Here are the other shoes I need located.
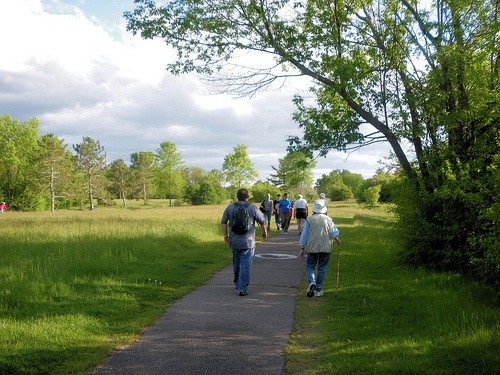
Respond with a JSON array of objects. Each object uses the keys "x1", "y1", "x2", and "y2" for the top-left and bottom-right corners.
[
  {"x1": 238, "y1": 291, "x2": 248, "y2": 296},
  {"x1": 305, "y1": 282, "x2": 316, "y2": 298},
  {"x1": 235, "y1": 287, "x2": 238, "y2": 290},
  {"x1": 315, "y1": 291, "x2": 323, "y2": 297}
]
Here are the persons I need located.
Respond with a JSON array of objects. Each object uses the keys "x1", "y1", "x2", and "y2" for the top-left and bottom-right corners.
[
  {"x1": 279, "y1": 193, "x2": 295, "y2": 235},
  {"x1": 294, "y1": 194, "x2": 309, "y2": 234},
  {"x1": 273, "y1": 194, "x2": 282, "y2": 230},
  {"x1": 319, "y1": 193, "x2": 328, "y2": 208},
  {"x1": 261, "y1": 193, "x2": 274, "y2": 231},
  {"x1": 221, "y1": 188, "x2": 268, "y2": 295},
  {"x1": 300, "y1": 199, "x2": 341, "y2": 298},
  {"x1": 0, "y1": 201, "x2": 7, "y2": 213}
]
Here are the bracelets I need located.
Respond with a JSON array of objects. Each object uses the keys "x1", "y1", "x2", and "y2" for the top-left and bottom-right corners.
[
  {"x1": 223, "y1": 235, "x2": 228, "y2": 237},
  {"x1": 301, "y1": 248, "x2": 304, "y2": 249}
]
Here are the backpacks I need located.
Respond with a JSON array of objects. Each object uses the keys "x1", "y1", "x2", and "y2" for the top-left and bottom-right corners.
[{"x1": 230, "y1": 201, "x2": 252, "y2": 235}]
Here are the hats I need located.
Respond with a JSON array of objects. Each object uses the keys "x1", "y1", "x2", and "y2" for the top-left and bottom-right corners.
[
  {"x1": 319, "y1": 192, "x2": 325, "y2": 198},
  {"x1": 311, "y1": 200, "x2": 327, "y2": 213}
]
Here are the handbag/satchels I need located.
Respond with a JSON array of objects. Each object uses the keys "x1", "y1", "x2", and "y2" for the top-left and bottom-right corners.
[{"x1": 259, "y1": 200, "x2": 266, "y2": 212}]
